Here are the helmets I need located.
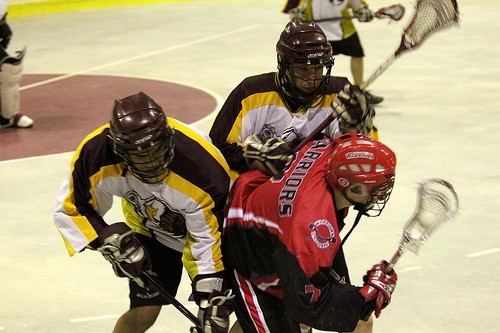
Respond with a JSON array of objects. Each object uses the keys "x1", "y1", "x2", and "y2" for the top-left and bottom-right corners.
[
  {"x1": 275, "y1": 21, "x2": 334, "y2": 109},
  {"x1": 325, "y1": 131, "x2": 397, "y2": 217},
  {"x1": 108, "y1": 91, "x2": 175, "y2": 183}
]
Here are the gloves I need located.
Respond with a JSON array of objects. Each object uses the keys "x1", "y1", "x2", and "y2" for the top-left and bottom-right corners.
[
  {"x1": 357, "y1": 6, "x2": 374, "y2": 21},
  {"x1": 99, "y1": 221, "x2": 152, "y2": 278},
  {"x1": 361, "y1": 259, "x2": 397, "y2": 318},
  {"x1": 190, "y1": 270, "x2": 236, "y2": 332},
  {"x1": 244, "y1": 133, "x2": 291, "y2": 179}
]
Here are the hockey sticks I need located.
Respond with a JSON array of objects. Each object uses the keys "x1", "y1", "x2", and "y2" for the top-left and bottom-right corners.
[
  {"x1": 289, "y1": 0, "x2": 459, "y2": 154},
  {"x1": 312, "y1": 4, "x2": 404, "y2": 22},
  {"x1": 140, "y1": 271, "x2": 201, "y2": 327},
  {"x1": 385, "y1": 177, "x2": 460, "y2": 273}
]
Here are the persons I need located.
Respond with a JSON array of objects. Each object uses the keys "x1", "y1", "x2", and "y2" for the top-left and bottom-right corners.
[
  {"x1": 220, "y1": 134, "x2": 397, "y2": 333},
  {"x1": 281, "y1": 0, "x2": 384, "y2": 104},
  {"x1": 0, "y1": 0, "x2": 34, "y2": 128},
  {"x1": 209, "y1": 18, "x2": 379, "y2": 333},
  {"x1": 53, "y1": 92, "x2": 240, "y2": 333}
]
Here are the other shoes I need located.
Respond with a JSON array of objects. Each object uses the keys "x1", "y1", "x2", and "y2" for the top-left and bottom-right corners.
[
  {"x1": 13, "y1": 113, "x2": 34, "y2": 126},
  {"x1": 365, "y1": 90, "x2": 384, "y2": 103}
]
